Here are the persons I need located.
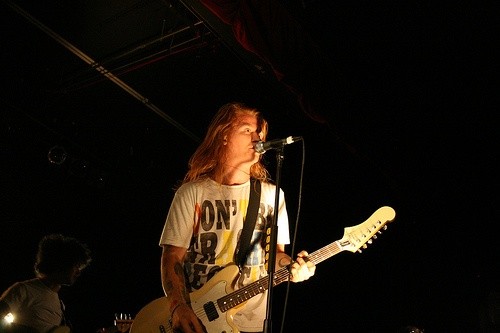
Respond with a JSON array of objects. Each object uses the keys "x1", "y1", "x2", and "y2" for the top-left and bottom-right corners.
[
  {"x1": 158, "y1": 101, "x2": 316, "y2": 333},
  {"x1": 0, "y1": 234, "x2": 92, "y2": 333}
]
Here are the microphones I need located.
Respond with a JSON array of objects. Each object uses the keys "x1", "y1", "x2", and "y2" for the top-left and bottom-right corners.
[{"x1": 254, "y1": 137, "x2": 300, "y2": 154}]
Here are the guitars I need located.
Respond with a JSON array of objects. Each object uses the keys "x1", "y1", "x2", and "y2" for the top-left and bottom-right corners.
[
  {"x1": 50, "y1": 312, "x2": 134, "y2": 333},
  {"x1": 129, "y1": 205, "x2": 397, "y2": 333}
]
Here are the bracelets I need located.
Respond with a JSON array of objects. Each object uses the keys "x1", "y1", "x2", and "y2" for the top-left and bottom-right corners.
[{"x1": 168, "y1": 302, "x2": 188, "y2": 320}]
